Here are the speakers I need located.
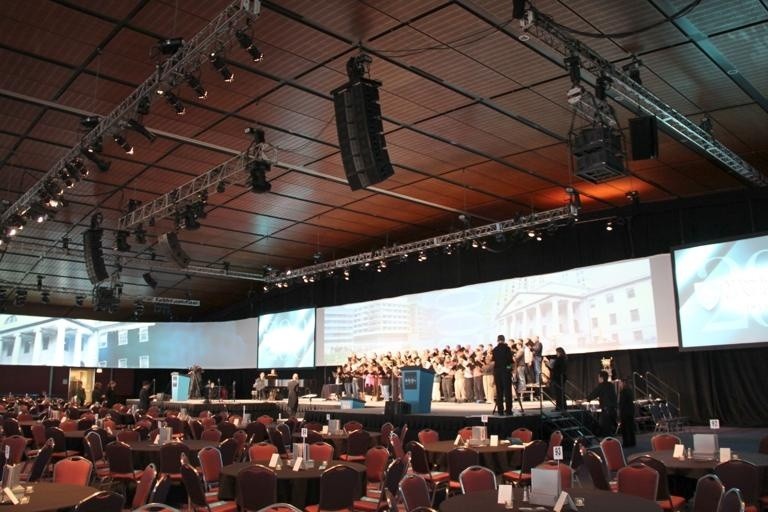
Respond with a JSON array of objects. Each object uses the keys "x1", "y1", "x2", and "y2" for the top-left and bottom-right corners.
[
  {"x1": 628, "y1": 115, "x2": 659, "y2": 160},
  {"x1": 385, "y1": 401, "x2": 411, "y2": 415}
]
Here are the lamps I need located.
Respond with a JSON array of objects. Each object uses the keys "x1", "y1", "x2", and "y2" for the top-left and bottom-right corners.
[{"x1": 260, "y1": 206, "x2": 580, "y2": 294}]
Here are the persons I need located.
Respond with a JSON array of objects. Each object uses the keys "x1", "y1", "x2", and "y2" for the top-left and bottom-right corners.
[
  {"x1": 138, "y1": 380, "x2": 157, "y2": 411},
  {"x1": 285, "y1": 373, "x2": 304, "y2": 417},
  {"x1": 615, "y1": 376, "x2": 637, "y2": 449},
  {"x1": 574, "y1": 370, "x2": 619, "y2": 441},
  {"x1": 252, "y1": 371, "x2": 268, "y2": 400},
  {"x1": 545, "y1": 347, "x2": 570, "y2": 412},
  {"x1": 76, "y1": 380, "x2": 86, "y2": 406},
  {"x1": 104, "y1": 379, "x2": 118, "y2": 409},
  {"x1": 92, "y1": 381, "x2": 106, "y2": 406},
  {"x1": 332, "y1": 333, "x2": 544, "y2": 417}
]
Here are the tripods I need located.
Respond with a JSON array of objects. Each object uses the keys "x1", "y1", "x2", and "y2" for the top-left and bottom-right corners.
[{"x1": 189, "y1": 374, "x2": 201, "y2": 398}]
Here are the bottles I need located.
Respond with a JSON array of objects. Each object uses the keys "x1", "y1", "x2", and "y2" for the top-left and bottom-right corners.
[
  {"x1": 687, "y1": 448, "x2": 691, "y2": 459},
  {"x1": 523, "y1": 485, "x2": 531, "y2": 501}
]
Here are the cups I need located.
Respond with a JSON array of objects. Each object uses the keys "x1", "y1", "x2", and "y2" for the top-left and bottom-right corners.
[
  {"x1": 575, "y1": 497, "x2": 585, "y2": 505},
  {"x1": 609, "y1": 480, "x2": 619, "y2": 494},
  {"x1": 505, "y1": 499, "x2": 514, "y2": 509}
]
